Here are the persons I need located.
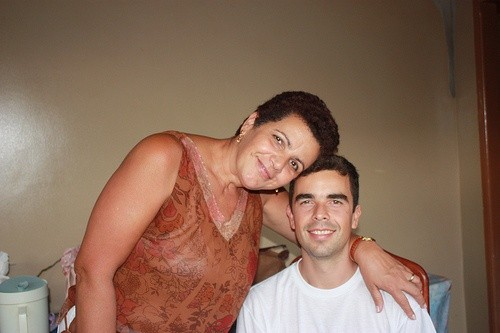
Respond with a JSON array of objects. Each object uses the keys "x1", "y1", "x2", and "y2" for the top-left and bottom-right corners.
[
  {"x1": 58, "y1": 91, "x2": 428, "y2": 333},
  {"x1": 235, "y1": 153, "x2": 438, "y2": 333}
]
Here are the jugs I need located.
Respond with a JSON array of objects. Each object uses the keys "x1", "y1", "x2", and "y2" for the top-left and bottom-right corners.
[{"x1": 0, "y1": 276, "x2": 49, "y2": 333}]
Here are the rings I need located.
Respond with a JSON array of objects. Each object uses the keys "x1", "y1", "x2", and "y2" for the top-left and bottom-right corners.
[{"x1": 409, "y1": 273, "x2": 415, "y2": 282}]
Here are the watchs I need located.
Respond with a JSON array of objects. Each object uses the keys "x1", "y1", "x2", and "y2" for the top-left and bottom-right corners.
[{"x1": 349, "y1": 235, "x2": 377, "y2": 262}]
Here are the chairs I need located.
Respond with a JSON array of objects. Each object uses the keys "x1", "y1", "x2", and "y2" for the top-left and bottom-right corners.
[{"x1": 290, "y1": 249, "x2": 430, "y2": 315}]
